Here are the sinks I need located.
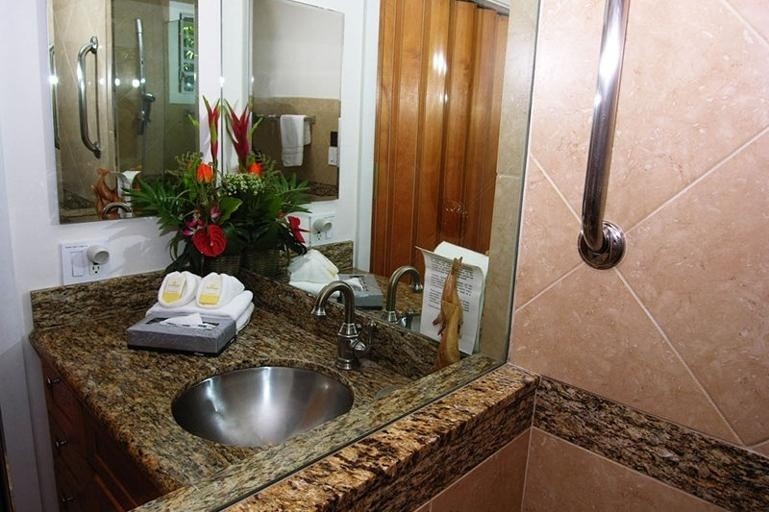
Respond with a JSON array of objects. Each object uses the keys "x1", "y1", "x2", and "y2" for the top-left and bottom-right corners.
[
  {"x1": 171, "y1": 361, "x2": 353, "y2": 448},
  {"x1": 397, "y1": 314, "x2": 425, "y2": 333}
]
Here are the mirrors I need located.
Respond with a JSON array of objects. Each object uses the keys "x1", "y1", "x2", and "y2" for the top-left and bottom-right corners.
[
  {"x1": 46, "y1": 1, "x2": 200, "y2": 225},
  {"x1": 220, "y1": 0, "x2": 544, "y2": 361},
  {"x1": 243, "y1": 2, "x2": 343, "y2": 207}
]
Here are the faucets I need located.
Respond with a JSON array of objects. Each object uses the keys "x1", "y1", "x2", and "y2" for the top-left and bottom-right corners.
[
  {"x1": 101, "y1": 203, "x2": 132, "y2": 219},
  {"x1": 379, "y1": 266, "x2": 424, "y2": 330},
  {"x1": 311, "y1": 280, "x2": 376, "y2": 372}
]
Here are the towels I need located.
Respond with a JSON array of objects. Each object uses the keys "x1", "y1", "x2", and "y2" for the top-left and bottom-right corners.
[
  {"x1": 146, "y1": 273, "x2": 255, "y2": 337},
  {"x1": 290, "y1": 250, "x2": 343, "y2": 303},
  {"x1": 276, "y1": 113, "x2": 311, "y2": 169}
]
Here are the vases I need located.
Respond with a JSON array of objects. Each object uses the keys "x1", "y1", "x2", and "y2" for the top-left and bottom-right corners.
[
  {"x1": 245, "y1": 245, "x2": 287, "y2": 277},
  {"x1": 184, "y1": 245, "x2": 242, "y2": 281}
]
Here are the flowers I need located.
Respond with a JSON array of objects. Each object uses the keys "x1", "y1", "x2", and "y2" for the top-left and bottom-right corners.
[
  {"x1": 121, "y1": 94, "x2": 247, "y2": 272},
  {"x1": 222, "y1": 102, "x2": 312, "y2": 258}
]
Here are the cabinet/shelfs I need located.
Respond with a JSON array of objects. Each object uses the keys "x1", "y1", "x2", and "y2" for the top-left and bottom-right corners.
[{"x1": 40, "y1": 359, "x2": 162, "y2": 511}]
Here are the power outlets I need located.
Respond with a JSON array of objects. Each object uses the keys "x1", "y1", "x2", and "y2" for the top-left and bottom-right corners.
[
  {"x1": 86, "y1": 238, "x2": 111, "y2": 281},
  {"x1": 308, "y1": 217, "x2": 326, "y2": 244}
]
